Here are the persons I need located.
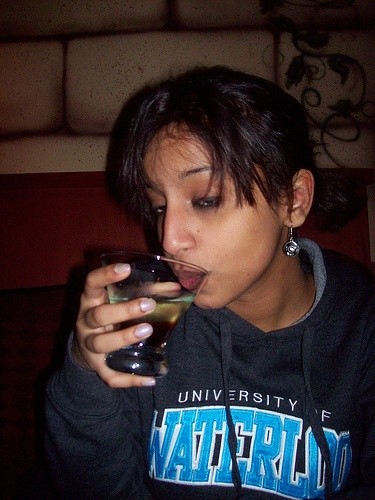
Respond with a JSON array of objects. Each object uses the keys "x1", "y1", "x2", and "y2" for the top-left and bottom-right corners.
[{"x1": 42, "y1": 60, "x2": 375, "y2": 500}]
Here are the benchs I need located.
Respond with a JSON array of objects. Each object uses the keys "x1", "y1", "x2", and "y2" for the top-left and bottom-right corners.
[{"x1": 1, "y1": 166, "x2": 375, "y2": 500}]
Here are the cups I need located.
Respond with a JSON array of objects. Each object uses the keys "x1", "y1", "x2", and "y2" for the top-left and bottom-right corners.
[{"x1": 98, "y1": 251, "x2": 209, "y2": 379}]
